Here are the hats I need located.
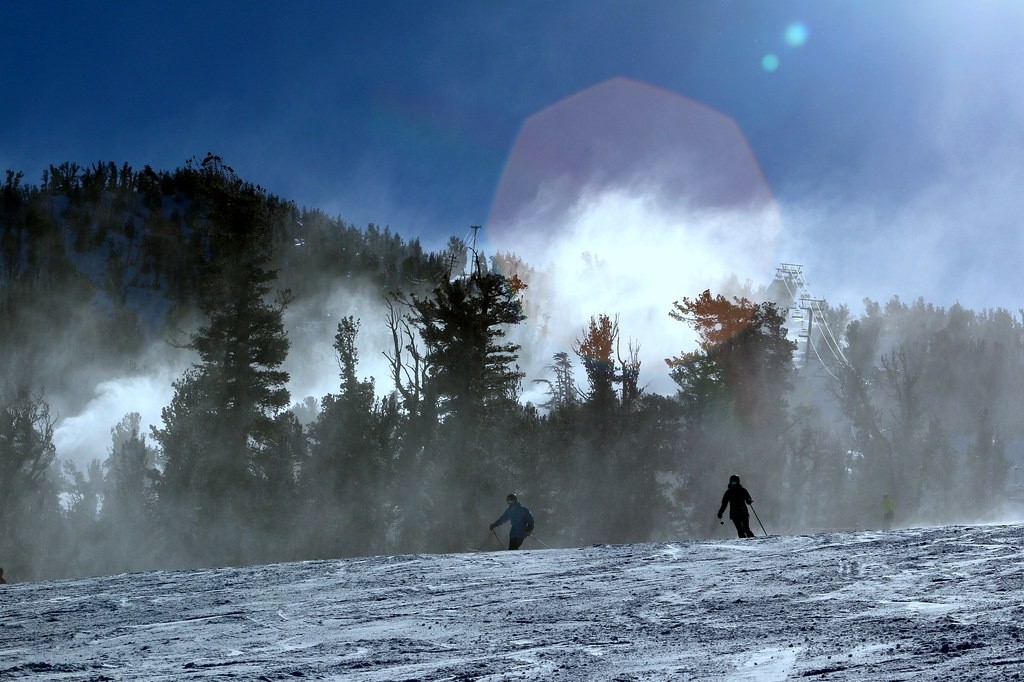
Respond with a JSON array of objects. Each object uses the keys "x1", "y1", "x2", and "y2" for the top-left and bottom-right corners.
[
  {"x1": 730, "y1": 475, "x2": 740, "y2": 485},
  {"x1": 507, "y1": 494, "x2": 517, "y2": 501}
]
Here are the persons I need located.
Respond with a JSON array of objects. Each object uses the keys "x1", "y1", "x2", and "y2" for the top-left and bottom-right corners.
[
  {"x1": 718, "y1": 474, "x2": 754, "y2": 538},
  {"x1": 490, "y1": 494, "x2": 535, "y2": 550},
  {"x1": 881, "y1": 493, "x2": 895, "y2": 531},
  {"x1": 0, "y1": 568, "x2": 6, "y2": 584}
]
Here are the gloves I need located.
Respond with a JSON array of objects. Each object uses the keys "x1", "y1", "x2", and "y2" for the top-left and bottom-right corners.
[
  {"x1": 489, "y1": 523, "x2": 497, "y2": 531},
  {"x1": 746, "y1": 499, "x2": 754, "y2": 505},
  {"x1": 527, "y1": 530, "x2": 531, "y2": 536},
  {"x1": 718, "y1": 513, "x2": 722, "y2": 519}
]
